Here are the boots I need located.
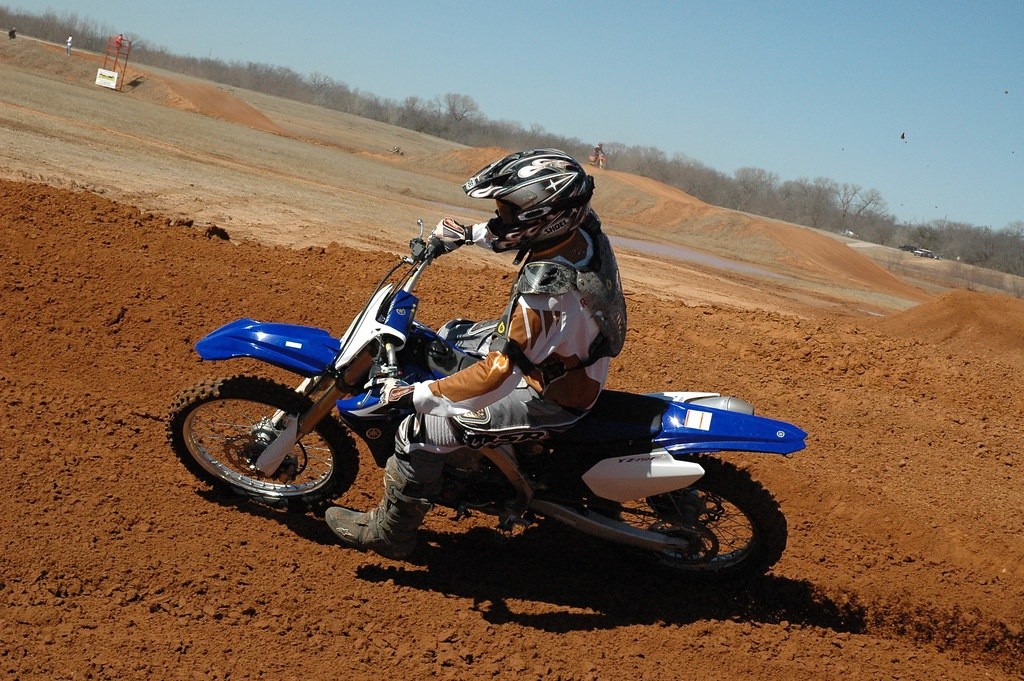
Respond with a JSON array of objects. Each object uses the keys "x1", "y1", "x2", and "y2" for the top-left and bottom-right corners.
[{"x1": 325, "y1": 454, "x2": 431, "y2": 552}]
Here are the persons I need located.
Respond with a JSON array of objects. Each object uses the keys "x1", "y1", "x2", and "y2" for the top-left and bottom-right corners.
[
  {"x1": 592, "y1": 142, "x2": 604, "y2": 165},
  {"x1": 66, "y1": 34, "x2": 73, "y2": 54},
  {"x1": 8, "y1": 26, "x2": 17, "y2": 40},
  {"x1": 116, "y1": 34, "x2": 124, "y2": 47},
  {"x1": 325, "y1": 149, "x2": 628, "y2": 548}
]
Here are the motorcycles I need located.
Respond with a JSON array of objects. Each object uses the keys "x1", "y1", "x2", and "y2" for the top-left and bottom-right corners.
[
  {"x1": 163, "y1": 220, "x2": 808, "y2": 590},
  {"x1": 589, "y1": 153, "x2": 608, "y2": 170}
]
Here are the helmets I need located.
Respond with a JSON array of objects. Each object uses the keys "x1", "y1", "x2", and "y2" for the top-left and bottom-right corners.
[{"x1": 462, "y1": 148, "x2": 593, "y2": 253}]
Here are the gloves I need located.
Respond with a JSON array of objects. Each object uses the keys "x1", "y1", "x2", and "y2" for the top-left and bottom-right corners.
[
  {"x1": 427, "y1": 217, "x2": 474, "y2": 254},
  {"x1": 363, "y1": 377, "x2": 414, "y2": 410}
]
